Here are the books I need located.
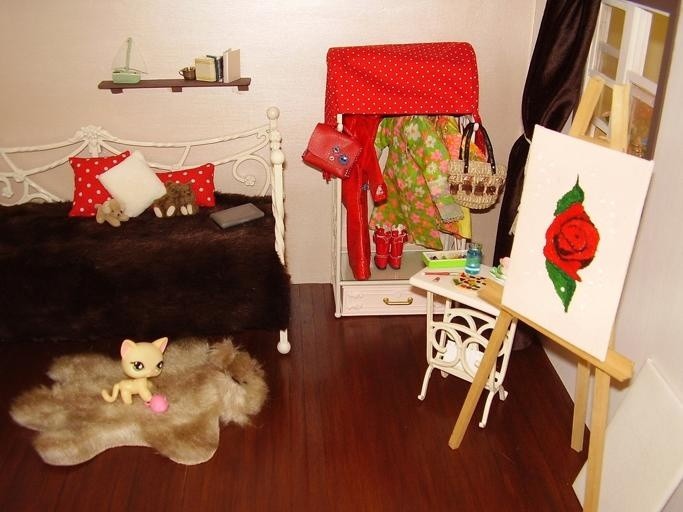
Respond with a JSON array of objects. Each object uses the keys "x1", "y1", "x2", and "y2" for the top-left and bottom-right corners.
[
  {"x1": 194, "y1": 57, "x2": 216, "y2": 83},
  {"x1": 222, "y1": 47, "x2": 241, "y2": 84},
  {"x1": 206, "y1": 55, "x2": 223, "y2": 83}
]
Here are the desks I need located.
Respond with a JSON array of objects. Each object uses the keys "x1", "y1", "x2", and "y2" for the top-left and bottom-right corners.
[{"x1": 407, "y1": 263, "x2": 519, "y2": 428}]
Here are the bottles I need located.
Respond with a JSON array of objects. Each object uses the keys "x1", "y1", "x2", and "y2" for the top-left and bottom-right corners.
[{"x1": 465, "y1": 242, "x2": 482, "y2": 274}]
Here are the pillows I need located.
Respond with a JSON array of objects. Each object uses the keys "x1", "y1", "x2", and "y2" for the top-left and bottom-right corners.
[{"x1": 68, "y1": 149, "x2": 215, "y2": 218}]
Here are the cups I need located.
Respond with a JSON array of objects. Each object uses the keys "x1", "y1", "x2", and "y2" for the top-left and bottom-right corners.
[{"x1": 178, "y1": 66, "x2": 196, "y2": 80}]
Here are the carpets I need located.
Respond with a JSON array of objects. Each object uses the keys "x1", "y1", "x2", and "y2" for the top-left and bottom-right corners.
[{"x1": 6, "y1": 336, "x2": 269, "y2": 467}]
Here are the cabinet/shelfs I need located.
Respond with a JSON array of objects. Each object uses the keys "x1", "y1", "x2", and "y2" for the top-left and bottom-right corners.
[{"x1": 329, "y1": 114, "x2": 479, "y2": 318}]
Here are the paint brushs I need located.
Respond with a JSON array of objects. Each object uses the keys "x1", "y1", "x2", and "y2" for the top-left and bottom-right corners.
[{"x1": 424, "y1": 272, "x2": 461, "y2": 276}]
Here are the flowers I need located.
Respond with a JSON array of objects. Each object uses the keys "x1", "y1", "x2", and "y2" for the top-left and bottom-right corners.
[{"x1": 543, "y1": 173, "x2": 600, "y2": 313}]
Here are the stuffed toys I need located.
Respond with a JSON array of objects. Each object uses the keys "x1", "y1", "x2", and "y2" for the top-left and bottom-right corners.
[
  {"x1": 93, "y1": 198, "x2": 130, "y2": 228},
  {"x1": 152, "y1": 180, "x2": 181, "y2": 218},
  {"x1": 174, "y1": 182, "x2": 199, "y2": 216}
]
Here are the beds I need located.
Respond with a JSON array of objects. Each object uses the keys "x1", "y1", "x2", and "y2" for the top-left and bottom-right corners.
[{"x1": 0, "y1": 107, "x2": 293, "y2": 354}]
